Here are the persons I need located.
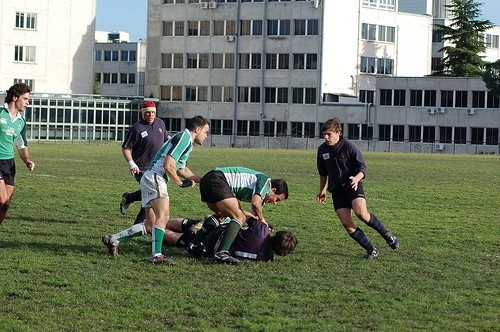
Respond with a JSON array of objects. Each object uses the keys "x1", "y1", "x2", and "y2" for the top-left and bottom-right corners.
[
  {"x1": 317, "y1": 119, "x2": 397, "y2": 258},
  {"x1": 164, "y1": 211, "x2": 298, "y2": 263},
  {"x1": 199, "y1": 167, "x2": 288, "y2": 265},
  {"x1": 0, "y1": 83, "x2": 36, "y2": 225},
  {"x1": 120, "y1": 101, "x2": 172, "y2": 224},
  {"x1": 103, "y1": 115, "x2": 211, "y2": 264}
]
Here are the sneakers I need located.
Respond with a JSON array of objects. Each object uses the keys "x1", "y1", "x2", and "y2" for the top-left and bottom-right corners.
[
  {"x1": 366, "y1": 247, "x2": 377, "y2": 258},
  {"x1": 103, "y1": 235, "x2": 119, "y2": 256},
  {"x1": 213, "y1": 252, "x2": 240, "y2": 264},
  {"x1": 187, "y1": 242, "x2": 212, "y2": 257},
  {"x1": 386, "y1": 234, "x2": 399, "y2": 249},
  {"x1": 120, "y1": 193, "x2": 130, "y2": 214},
  {"x1": 149, "y1": 255, "x2": 176, "y2": 266}
]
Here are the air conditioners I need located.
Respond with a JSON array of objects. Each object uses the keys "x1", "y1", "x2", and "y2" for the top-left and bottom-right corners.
[
  {"x1": 200, "y1": 2, "x2": 208, "y2": 8},
  {"x1": 427, "y1": 108, "x2": 436, "y2": 114},
  {"x1": 209, "y1": 2, "x2": 217, "y2": 8},
  {"x1": 225, "y1": 36, "x2": 235, "y2": 42},
  {"x1": 465, "y1": 108, "x2": 475, "y2": 115},
  {"x1": 310, "y1": 0, "x2": 319, "y2": 8},
  {"x1": 437, "y1": 107, "x2": 446, "y2": 113},
  {"x1": 435, "y1": 144, "x2": 444, "y2": 150}
]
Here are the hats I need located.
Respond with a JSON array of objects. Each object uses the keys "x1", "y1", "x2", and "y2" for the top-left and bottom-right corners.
[{"x1": 140, "y1": 102, "x2": 156, "y2": 120}]
